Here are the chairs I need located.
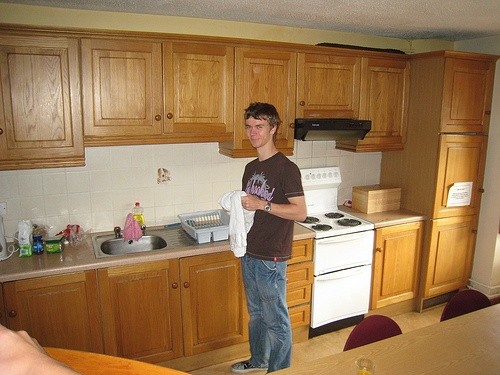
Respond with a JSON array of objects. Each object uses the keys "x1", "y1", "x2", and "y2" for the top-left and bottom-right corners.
[
  {"x1": 440, "y1": 289, "x2": 491, "y2": 322},
  {"x1": 343, "y1": 314, "x2": 403, "y2": 352}
]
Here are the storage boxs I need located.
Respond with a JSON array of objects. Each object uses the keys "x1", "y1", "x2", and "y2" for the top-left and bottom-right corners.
[{"x1": 352, "y1": 184, "x2": 401, "y2": 215}]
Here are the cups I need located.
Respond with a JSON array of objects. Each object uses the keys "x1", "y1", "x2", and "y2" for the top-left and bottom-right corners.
[{"x1": 355, "y1": 358, "x2": 375, "y2": 375}]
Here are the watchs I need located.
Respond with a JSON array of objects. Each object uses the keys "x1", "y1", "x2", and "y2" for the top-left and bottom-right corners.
[{"x1": 264, "y1": 202, "x2": 272, "y2": 212}]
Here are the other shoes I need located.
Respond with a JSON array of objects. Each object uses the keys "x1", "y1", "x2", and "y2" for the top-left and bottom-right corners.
[{"x1": 231, "y1": 361, "x2": 269, "y2": 372}]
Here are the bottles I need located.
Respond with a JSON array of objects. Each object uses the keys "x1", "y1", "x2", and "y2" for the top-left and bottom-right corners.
[
  {"x1": 32, "y1": 225, "x2": 44, "y2": 254},
  {"x1": 131, "y1": 202, "x2": 144, "y2": 226}
]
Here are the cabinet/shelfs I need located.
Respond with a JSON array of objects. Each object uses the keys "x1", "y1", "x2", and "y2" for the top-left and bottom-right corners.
[
  {"x1": 417, "y1": 214, "x2": 479, "y2": 312},
  {"x1": 380, "y1": 132, "x2": 489, "y2": 218},
  {"x1": 0, "y1": 268, "x2": 102, "y2": 356},
  {"x1": 371, "y1": 221, "x2": 426, "y2": 311},
  {"x1": 77, "y1": 26, "x2": 234, "y2": 147},
  {"x1": 219, "y1": 37, "x2": 295, "y2": 159},
  {"x1": 297, "y1": 45, "x2": 359, "y2": 121},
  {"x1": 335, "y1": 44, "x2": 409, "y2": 153},
  {"x1": 97, "y1": 252, "x2": 251, "y2": 372},
  {"x1": 407, "y1": 50, "x2": 500, "y2": 132},
  {"x1": 0, "y1": 21, "x2": 86, "y2": 171},
  {"x1": 285, "y1": 236, "x2": 315, "y2": 343}
]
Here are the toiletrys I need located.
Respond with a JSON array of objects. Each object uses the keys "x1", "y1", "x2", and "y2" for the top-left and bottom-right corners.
[{"x1": 131, "y1": 201, "x2": 145, "y2": 226}]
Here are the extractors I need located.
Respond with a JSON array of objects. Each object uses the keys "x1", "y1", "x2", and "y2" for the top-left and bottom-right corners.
[{"x1": 295, "y1": 117, "x2": 372, "y2": 141}]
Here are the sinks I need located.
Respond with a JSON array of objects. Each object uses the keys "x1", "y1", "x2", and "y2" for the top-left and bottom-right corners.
[{"x1": 91, "y1": 226, "x2": 175, "y2": 259}]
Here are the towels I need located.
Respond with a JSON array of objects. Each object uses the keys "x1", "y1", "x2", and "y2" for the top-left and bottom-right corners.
[
  {"x1": 216, "y1": 190, "x2": 256, "y2": 258},
  {"x1": 122, "y1": 213, "x2": 144, "y2": 241}
]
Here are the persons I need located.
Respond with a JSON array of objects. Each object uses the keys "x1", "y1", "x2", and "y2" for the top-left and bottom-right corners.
[
  {"x1": 231, "y1": 102, "x2": 307, "y2": 375},
  {"x1": 0, "y1": 323, "x2": 81, "y2": 375}
]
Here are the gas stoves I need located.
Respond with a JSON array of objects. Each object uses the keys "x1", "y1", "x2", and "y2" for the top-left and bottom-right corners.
[{"x1": 295, "y1": 167, "x2": 375, "y2": 239}]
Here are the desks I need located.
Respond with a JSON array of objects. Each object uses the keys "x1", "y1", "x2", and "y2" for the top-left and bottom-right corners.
[{"x1": 264, "y1": 304, "x2": 500, "y2": 375}]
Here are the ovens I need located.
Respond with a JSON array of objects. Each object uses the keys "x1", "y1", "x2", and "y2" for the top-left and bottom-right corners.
[{"x1": 309, "y1": 230, "x2": 375, "y2": 338}]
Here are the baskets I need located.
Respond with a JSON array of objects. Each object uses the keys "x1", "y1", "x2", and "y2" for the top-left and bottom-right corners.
[{"x1": 352, "y1": 186, "x2": 402, "y2": 214}]
